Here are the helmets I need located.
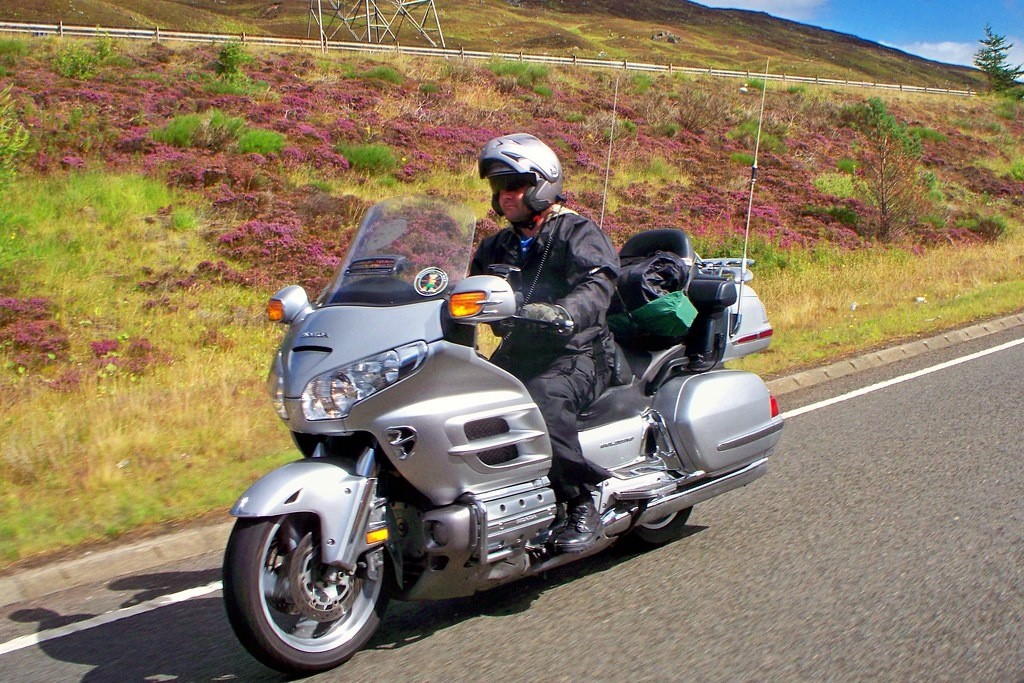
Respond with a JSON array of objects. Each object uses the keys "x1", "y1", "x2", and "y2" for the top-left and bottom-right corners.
[{"x1": 478, "y1": 132, "x2": 564, "y2": 218}]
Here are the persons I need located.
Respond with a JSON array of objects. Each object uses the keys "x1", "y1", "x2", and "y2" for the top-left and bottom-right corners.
[{"x1": 467, "y1": 133, "x2": 620, "y2": 554}]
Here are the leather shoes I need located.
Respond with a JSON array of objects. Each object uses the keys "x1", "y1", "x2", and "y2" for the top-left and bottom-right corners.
[{"x1": 554, "y1": 496, "x2": 606, "y2": 553}]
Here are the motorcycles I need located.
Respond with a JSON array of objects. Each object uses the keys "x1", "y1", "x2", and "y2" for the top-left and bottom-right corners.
[{"x1": 220, "y1": 195, "x2": 784, "y2": 679}]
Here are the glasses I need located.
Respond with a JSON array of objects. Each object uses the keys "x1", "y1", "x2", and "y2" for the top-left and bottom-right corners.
[{"x1": 488, "y1": 173, "x2": 547, "y2": 192}]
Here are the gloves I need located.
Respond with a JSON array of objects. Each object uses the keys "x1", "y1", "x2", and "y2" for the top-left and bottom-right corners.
[{"x1": 520, "y1": 302, "x2": 575, "y2": 337}]
